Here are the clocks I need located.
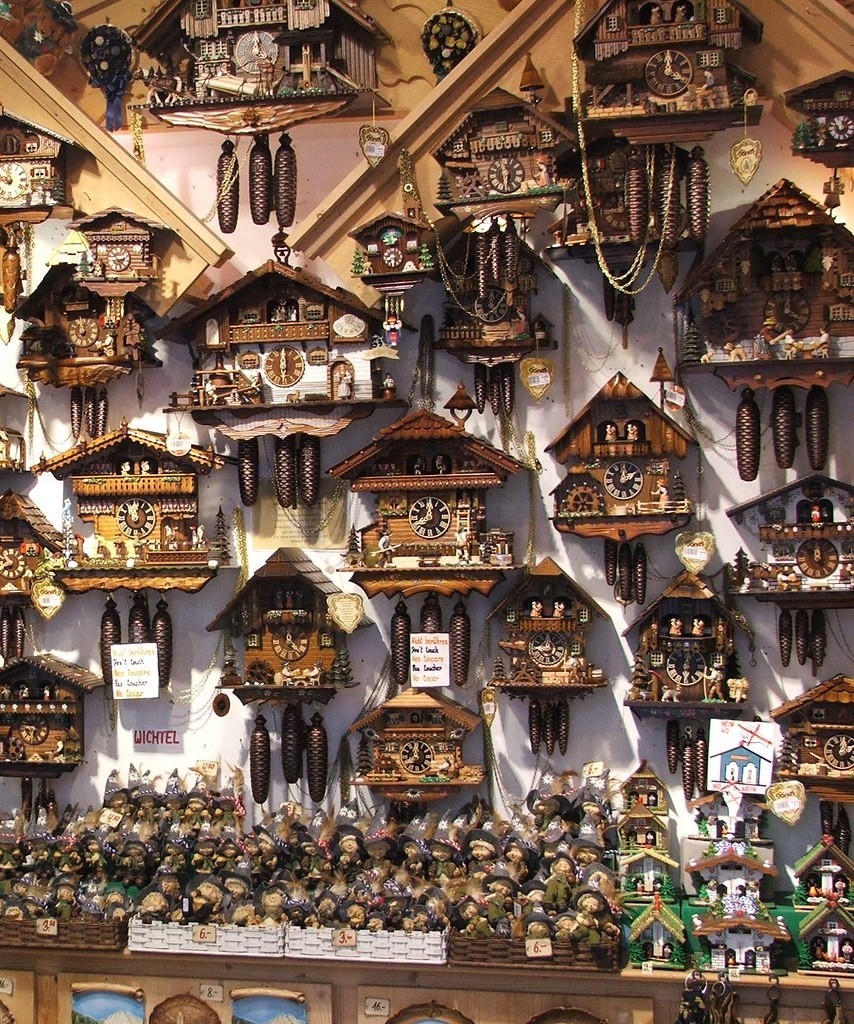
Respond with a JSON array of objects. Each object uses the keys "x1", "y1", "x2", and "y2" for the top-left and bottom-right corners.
[{"x1": 0, "y1": 0, "x2": 854, "y2": 858}]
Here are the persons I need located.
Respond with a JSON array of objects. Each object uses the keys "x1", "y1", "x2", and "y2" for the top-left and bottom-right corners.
[{"x1": 0, "y1": 0, "x2": 854, "y2": 980}]
